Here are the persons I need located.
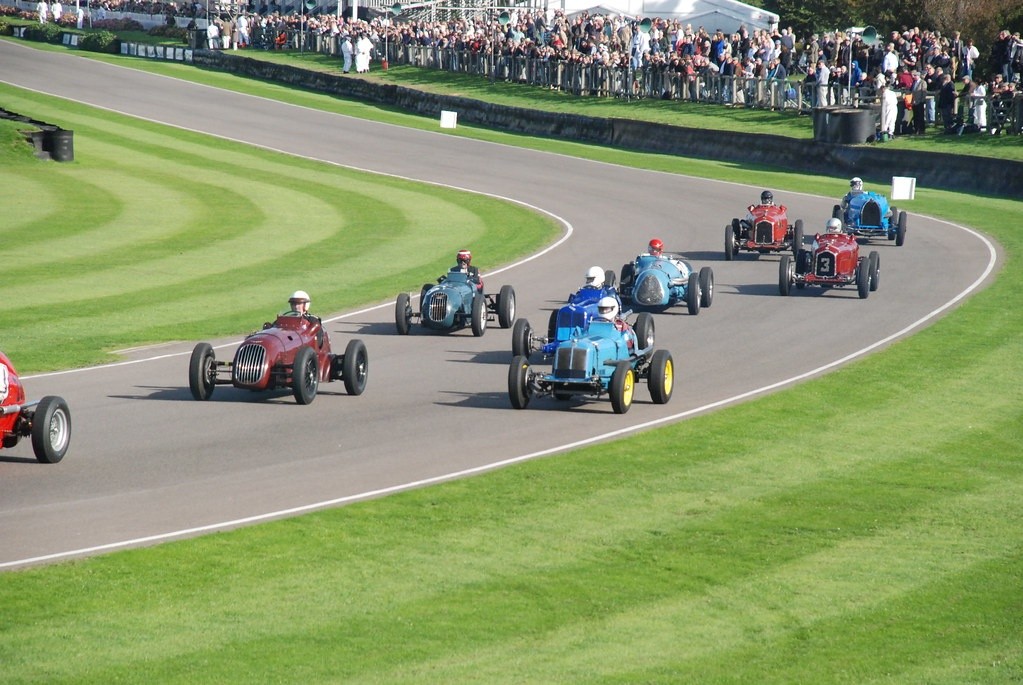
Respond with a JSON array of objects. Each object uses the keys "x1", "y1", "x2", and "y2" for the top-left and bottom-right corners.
[
  {"x1": 77, "y1": 6, "x2": 85, "y2": 30},
  {"x1": 826, "y1": 218, "x2": 842, "y2": 234},
  {"x1": 36, "y1": 0, "x2": 49, "y2": 24},
  {"x1": 841, "y1": 177, "x2": 868, "y2": 212},
  {"x1": 648, "y1": 239, "x2": 664, "y2": 257},
  {"x1": 598, "y1": 297, "x2": 633, "y2": 349},
  {"x1": 51, "y1": 0, "x2": 63, "y2": 25},
  {"x1": 451, "y1": 250, "x2": 483, "y2": 290},
  {"x1": 585, "y1": 266, "x2": 605, "y2": 290},
  {"x1": 760, "y1": 190, "x2": 776, "y2": 206},
  {"x1": 288, "y1": 290, "x2": 324, "y2": 349},
  {"x1": 64, "y1": 0, "x2": 1023, "y2": 140}
]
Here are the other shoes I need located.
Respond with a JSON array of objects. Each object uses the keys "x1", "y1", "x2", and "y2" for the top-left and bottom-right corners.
[{"x1": 344, "y1": 71, "x2": 350, "y2": 74}]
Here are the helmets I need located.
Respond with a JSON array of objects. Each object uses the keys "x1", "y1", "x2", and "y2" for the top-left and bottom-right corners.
[
  {"x1": 850, "y1": 177, "x2": 862, "y2": 191},
  {"x1": 288, "y1": 290, "x2": 311, "y2": 312},
  {"x1": 598, "y1": 296, "x2": 619, "y2": 320},
  {"x1": 585, "y1": 266, "x2": 605, "y2": 286},
  {"x1": 761, "y1": 191, "x2": 773, "y2": 204},
  {"x1": 457, "y1": 250, "x2": 472, "y2": 266},
  {"x1": 648, "y1": 239, "x2": 664, "y2": 256},
  {"x1": 826, "y1": 218, "x2": 842, "y2": 233}
]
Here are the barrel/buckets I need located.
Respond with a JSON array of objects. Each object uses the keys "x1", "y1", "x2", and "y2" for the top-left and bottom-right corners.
[{"x1": 880, "y1": 131, "x2": 889, "y2": 143}]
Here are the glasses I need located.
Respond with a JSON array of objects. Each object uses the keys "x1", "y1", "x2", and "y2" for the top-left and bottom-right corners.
[{"x1": 457, "y1": 259, "x2": 470, "y2": 263}]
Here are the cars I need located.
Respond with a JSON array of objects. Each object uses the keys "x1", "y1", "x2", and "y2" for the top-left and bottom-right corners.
[
  {"x1": 395, "y1": 271, "x2": 516, "y2": 338},
  {"x1": 508, "y1": 316, "x2": 675, "y2": 414},
  {"x1": 778, "y1": 232, "x2": 881, "y2": 299},
  {"x1": 189, "y1": 311, "x2": 369, "y2": 406},
  {"x1": 548, "y1": 285, "x2": 622, "y2": 345},
  {"x1": 601, "y1": 253, "x2": 714, "y2": 315},
  {"x1": 725, "y1": 204, "x2": 805, "y2": 262},
  {"x1": 512, "y1": 303, "x2": 656, "y2": 360},
  {"x1": 832, "y1": 190, "x2": 907, "y2": 246},
  {"x1": 0, "y1": 350, "x2": 72, "y2": 464}
]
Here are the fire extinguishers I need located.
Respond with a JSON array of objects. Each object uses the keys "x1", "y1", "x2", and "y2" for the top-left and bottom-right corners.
[{"x1": 382, "y1": 58, "x2": 386, "y2": 69}]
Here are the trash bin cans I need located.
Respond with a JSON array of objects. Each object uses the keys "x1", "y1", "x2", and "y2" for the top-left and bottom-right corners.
[{"x1": 190, "y1": 28, "x2": 208, "y2": 50}]
